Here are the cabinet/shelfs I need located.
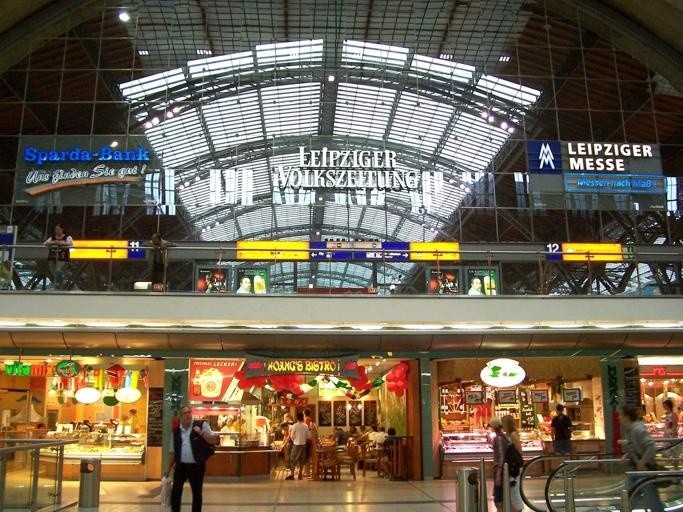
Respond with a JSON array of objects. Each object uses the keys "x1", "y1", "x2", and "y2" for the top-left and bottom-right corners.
[
  {"x1": 31, "y1": 403, "x2": 274, "y2": 483},
  {"x1": 438, "y1": 426, "x2": 606, "y2": 479}
]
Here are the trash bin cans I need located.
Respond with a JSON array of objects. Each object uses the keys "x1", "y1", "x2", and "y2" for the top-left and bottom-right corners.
[
  {"x1": 78, "y1": 457, "x2": 101, "y2": 508},
  {"x1": 456, "y1": 466, "x2": 478, "y2": 512}
]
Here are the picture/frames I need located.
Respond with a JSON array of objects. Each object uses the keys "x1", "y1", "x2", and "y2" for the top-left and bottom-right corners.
[{"x1": 461, "y1": 388, "x2": 581, "y2": 405}]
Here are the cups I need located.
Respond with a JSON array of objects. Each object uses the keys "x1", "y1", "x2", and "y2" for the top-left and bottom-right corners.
[
  {"x1": 252, "y1": 274, "x2": 265, "y2": 294},
  {"x1": 483, "y1": 275, "x2": 496, "y2": 295}
]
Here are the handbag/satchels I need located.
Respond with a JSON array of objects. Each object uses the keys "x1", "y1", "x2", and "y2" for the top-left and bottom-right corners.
[
  {"x1": 507, "y1": 444, "x2": 524, "y2": 468},
  {"x1": 649, "y1": 463, "x2": 673, "y2": 487},
  {"x1": 197, "y1": 436, "x2": 213, "y2": 459}
]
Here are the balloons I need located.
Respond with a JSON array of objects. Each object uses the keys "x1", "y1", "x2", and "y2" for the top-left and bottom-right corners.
[{"x1": 233, "y1": 361, "x2": 412, "y2": 398}]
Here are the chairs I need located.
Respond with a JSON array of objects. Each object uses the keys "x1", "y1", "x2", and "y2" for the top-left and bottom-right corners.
[{"x1": 310, "y1": 423, "x2": 414, "y2": 483}]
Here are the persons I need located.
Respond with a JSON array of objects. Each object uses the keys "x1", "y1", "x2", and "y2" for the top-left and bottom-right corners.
[
  {"x1": 487, "y1": 417, "x2": 510, "y2": 512},
  {"x1": 142, "y1": 233, "x2": 177, "y2": 290},
  {"x1": 663, "y1": 400, "x2": 681, "y2": 468},
  {"x1": 467, "y1": 277, "x2": 483, "y2": 295},
  {"x1": 278, "y1": 408, "x2": 313, "y2": 480},
  {"x1": 43, "y1": 223, "x2": 72, "y2": 287},
  {"x1": 219, "y1": 417, "x2": 239, "y2": 447},
  {"x1": 480, "y1": 421, "x2": 496, "y2": 442},
  {"x1": 127, "y1": 409, "x2": 138, "y2": 433},
  {"x1": 162, "y1": 405, "x2": 218, "y2": 512},
  {"x1": 252, "y1": 271, "x2": 266, "y2": 294},
  {"x1": 550, "y1": 404, "x2": 574, "y2": 477},
  {"x1": 501, "y1": 415, "x2": 524, "y2": 512},
  {"x1": 236, "y1": 276, "x2": 251, "y2": 294},
  {"x1": 329, "y1": 425, "x2": 396, "y2": 456},
  {"x1": 617, "y1": 405, "x2": 663, "y2": 512},
  {"x1": 482, "y1": 272, "x2": 497, "y2": 296}
]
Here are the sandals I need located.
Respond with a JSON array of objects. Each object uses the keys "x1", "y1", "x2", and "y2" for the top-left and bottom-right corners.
[{"x1": 285, "y1": 475, "x2": 295, "y2": 480}]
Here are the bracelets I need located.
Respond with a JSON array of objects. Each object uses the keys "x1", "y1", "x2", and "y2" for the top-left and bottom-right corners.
[
  {"x1": 166, "y1": 463, "x2": 173, "y2": 466},
  {"x1": 200, "y1": 430, "x2": 204, "y2": 436}
]
